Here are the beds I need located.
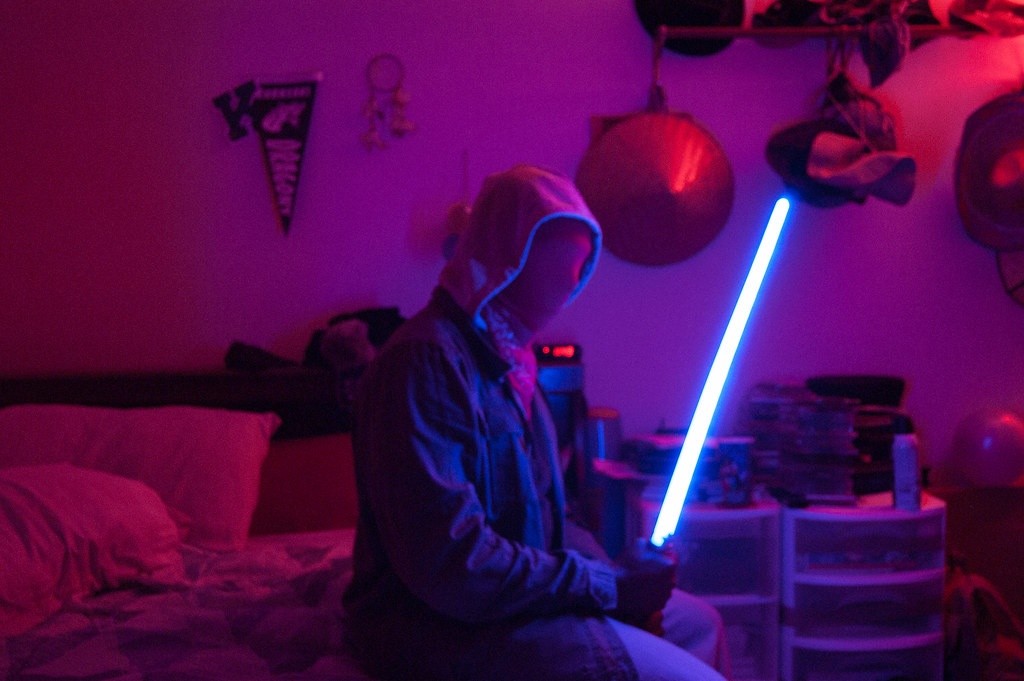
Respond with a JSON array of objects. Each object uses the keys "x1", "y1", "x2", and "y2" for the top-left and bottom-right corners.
[{"x1": 0, "y1": 363, "x2": 373, "y2": 681}]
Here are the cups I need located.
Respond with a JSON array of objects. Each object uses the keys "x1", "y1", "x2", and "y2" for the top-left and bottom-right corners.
[{"x1": 719, "y1": 441, "x2": 753, "y2": 504}]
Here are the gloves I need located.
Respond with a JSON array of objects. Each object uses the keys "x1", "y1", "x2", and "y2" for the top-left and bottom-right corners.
[
  {"x1": 636, "y1": 550, "x2": 676, "y2": 611},
  {"x1": 612, "y1": 566, "x2": 672, "y2": 621}
]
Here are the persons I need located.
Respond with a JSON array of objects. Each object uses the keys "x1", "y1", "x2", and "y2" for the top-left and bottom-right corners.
[{"x1": 347, "y1": 166, "x2": 730, "y2": 681}]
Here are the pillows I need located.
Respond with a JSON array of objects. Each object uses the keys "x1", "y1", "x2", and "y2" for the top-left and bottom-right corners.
[
  {"x1": 0, "y1": 403, "x2": 284, "y2": 554},
  {"x1": 0, "y1": 463, "x2": 184, "y2": 638}
]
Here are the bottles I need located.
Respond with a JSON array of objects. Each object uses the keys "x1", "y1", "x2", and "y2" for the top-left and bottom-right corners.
[{"x1": 891, "y1": 412, "x2": 922, "y2": 510}]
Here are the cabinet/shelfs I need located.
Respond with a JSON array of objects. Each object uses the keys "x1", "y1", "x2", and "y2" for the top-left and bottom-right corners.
[
  {"x1": 780, "y1": 486, "x2": 947, "y2": 681},
  {"x1": 624, "y1": 483, "x2": 780, "y2": 681}
]
